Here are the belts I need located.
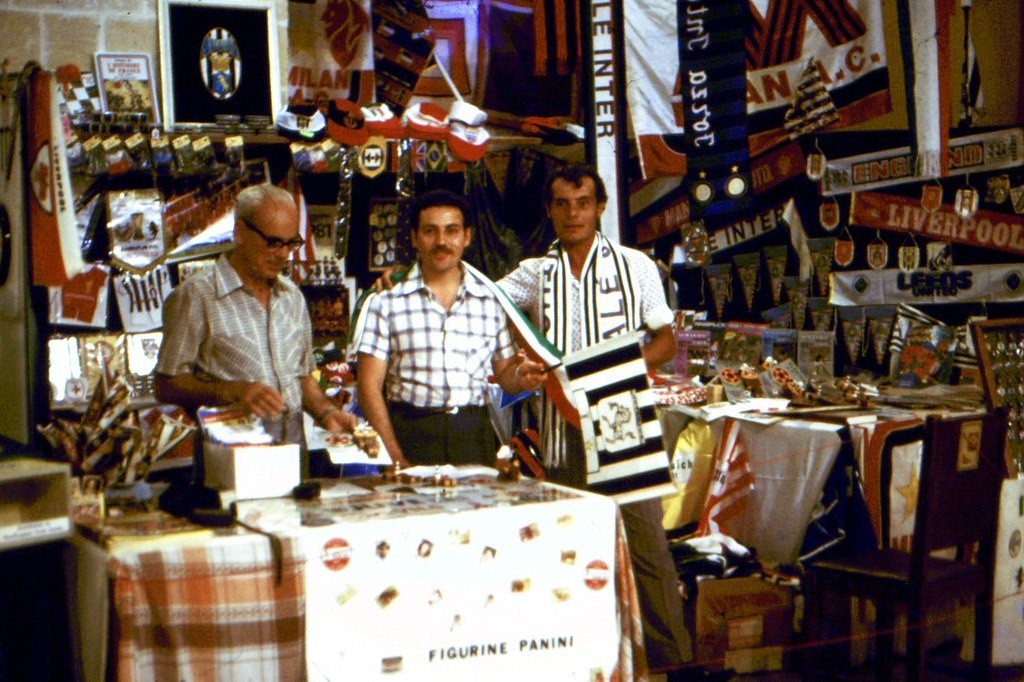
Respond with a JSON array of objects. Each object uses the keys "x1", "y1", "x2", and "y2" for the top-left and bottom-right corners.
[{"x1": 389, "y1": 400, "x2": 487, "y2": 418}]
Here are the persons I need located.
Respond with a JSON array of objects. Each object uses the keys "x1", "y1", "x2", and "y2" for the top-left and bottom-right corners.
[
  {"x1": 349, "y1": 189, "x2": 553, "y2": 470},
  {"x1": 154, "y1": 185, "x2": 359, "y2": 483},
  {"x1": 489, "y1": 163, "x2": 711, "y2": 681}
]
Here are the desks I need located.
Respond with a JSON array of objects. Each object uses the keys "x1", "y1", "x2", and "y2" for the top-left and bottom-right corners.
[{"x1": 67, "y1": 461, "x2": 650, "y2": 682}]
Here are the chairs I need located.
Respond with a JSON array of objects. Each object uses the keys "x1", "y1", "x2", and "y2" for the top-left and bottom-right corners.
[{"x1": 796, "y1": 403, "x2": 1011, "y2": 682}]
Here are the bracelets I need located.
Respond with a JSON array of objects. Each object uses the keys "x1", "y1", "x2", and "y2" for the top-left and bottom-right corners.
[{"x1": 314, "y1": 404, "x2": 340, "y2": 425}]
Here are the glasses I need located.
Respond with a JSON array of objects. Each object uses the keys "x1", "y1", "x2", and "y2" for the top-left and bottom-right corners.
[{"x1": 243, "y1": 220, "x2": 304, "y2": 250}]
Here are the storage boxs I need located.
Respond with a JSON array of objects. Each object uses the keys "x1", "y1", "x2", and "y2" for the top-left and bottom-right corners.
[{"x1": 679, "y1": 577, "x2": 794, "y2": 673}]
